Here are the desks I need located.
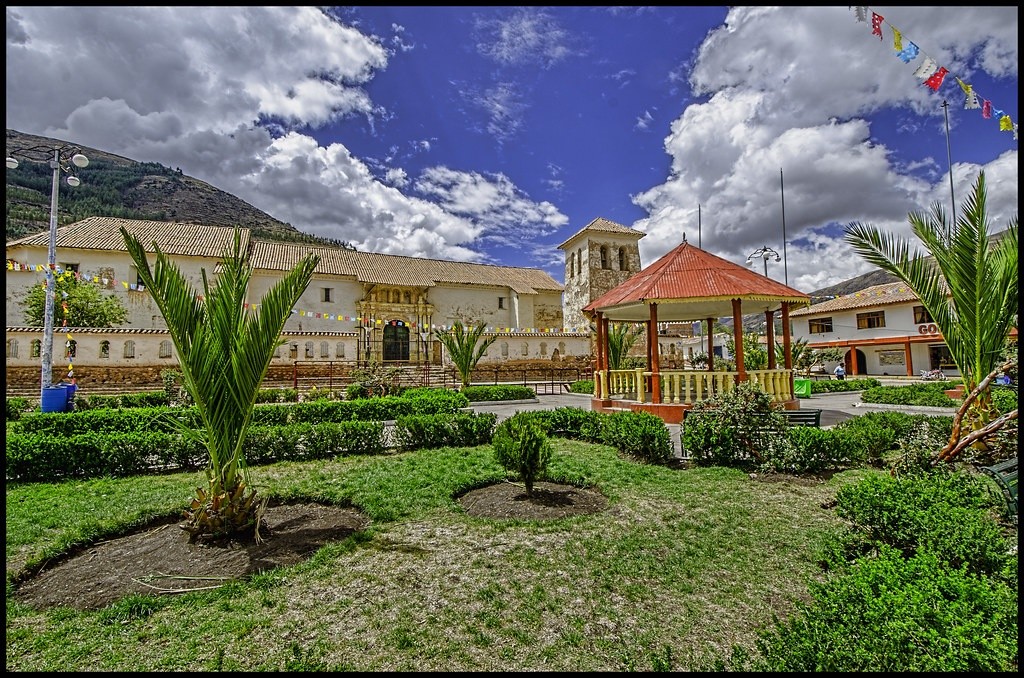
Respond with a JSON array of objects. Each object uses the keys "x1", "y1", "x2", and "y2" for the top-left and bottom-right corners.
[{"x1": 798, "y1": 373, "x2": 832, "y2": 381}]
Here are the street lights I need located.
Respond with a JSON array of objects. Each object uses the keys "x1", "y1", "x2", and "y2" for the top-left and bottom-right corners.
[
  {"x1": 745, "y1": 246, "x2": 781, "y2": 369},
  {"x1": 7, "y1": 145, "x2": 87, "y2": 410}
]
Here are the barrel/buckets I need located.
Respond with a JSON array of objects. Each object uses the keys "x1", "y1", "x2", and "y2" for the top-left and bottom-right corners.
[{"x1": 40, "y1": 381, "x2": 75, "y2": 414}]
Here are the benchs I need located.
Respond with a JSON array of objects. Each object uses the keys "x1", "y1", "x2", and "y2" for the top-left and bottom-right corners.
[
  {"x1": 680, "y1": 409, "x2": 823, "y2": 457},
  {"x1": 978, "y1": 456, "x2": 1019, "y2": 518}
]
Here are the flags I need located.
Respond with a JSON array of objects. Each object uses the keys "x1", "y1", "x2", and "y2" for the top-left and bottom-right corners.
[
  {"x1": 192, "y1": 294, "x2": 258, "y2": 312},
  {"x1": 62, "y1": 291, "x2": 78, "y2": 390},
  {"x1": 849, "y1": 7, "x2": 1018, "y2": 141},
  {"x1": 293, "y1": 310, "x2": 589, "y2": 334},
  {"x1": 614, "y1": 322, "x2": 647, "y2": 327},
  {"x1": 811, "y1": 289, "x2": 912, "y2": 300},
  {"x1": 6, "y1": 262, "x2": 145, "y2": 292}
]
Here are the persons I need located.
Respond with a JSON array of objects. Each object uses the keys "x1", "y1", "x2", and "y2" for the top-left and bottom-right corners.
[{"x1": 834, "y1": 363, "x2": 847, "y2": 381}]
